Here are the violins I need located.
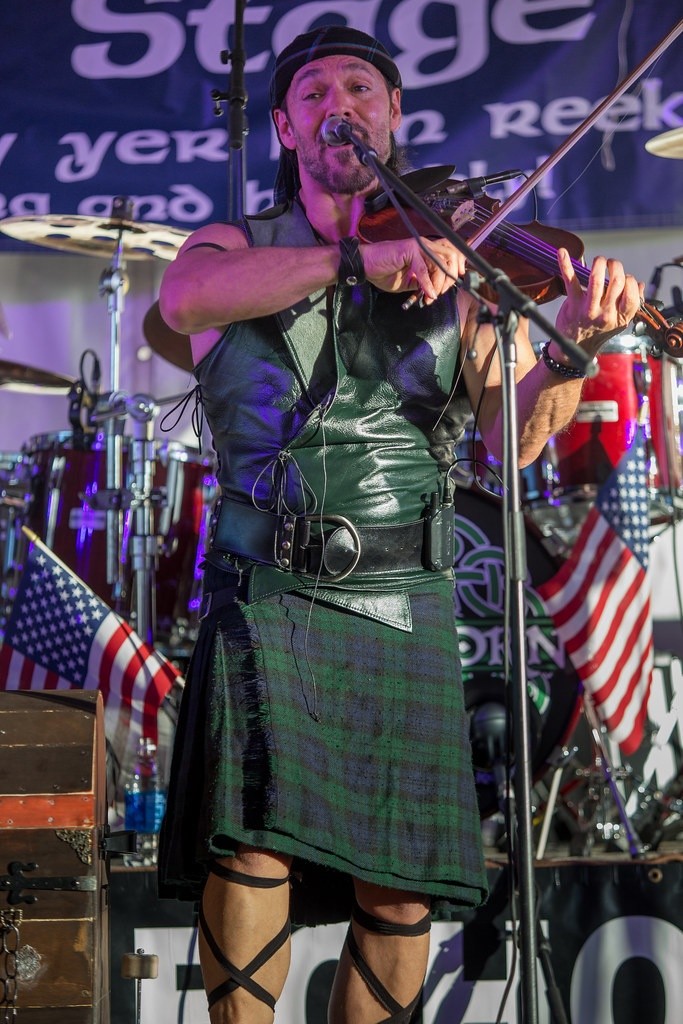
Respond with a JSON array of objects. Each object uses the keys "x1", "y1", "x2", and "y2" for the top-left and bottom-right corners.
[{"x1": 354, "y1": 178, "x2": 683, "y2": 358}]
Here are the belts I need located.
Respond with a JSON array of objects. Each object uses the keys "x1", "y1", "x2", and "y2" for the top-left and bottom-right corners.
[{"x1": 209, "y1": 495, "x2": 454, "y2": 581}]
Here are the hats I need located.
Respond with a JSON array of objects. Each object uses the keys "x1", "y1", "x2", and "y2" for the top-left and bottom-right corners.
[{"x1": 270, "y1": 26, "x2": 403, "y2": 109}]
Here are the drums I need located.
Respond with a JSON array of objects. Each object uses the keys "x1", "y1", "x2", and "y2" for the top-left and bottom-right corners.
[
  {"x1": 12, "y1": 425, "x2": 222, "y2": 636},
  {"x1": 516, "y1": 330, "x2": 683, "y2": 528},
  {"x1": 447, "y1": 479, "x2": 587, "y2": 821}
]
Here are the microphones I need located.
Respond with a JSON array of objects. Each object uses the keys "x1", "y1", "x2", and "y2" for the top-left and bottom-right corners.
[
  {"x1": 321, "y1": 116, "x2": 350, "y2": 147},
  {"x1": 80, "y1": 361, "x2": 101, "y2": 450}
]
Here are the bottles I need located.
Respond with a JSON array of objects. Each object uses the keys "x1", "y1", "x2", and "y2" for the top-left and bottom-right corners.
[{"x1": 125, "y1": 736, "x2": 165, "y2": 867}]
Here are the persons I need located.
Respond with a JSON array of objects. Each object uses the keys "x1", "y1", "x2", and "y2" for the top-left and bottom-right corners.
[{"x1": 157, "y1": 27, "x2": 647, "y2": 1024}]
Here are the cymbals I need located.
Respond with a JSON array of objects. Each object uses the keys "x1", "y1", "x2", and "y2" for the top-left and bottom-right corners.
[
  {"x1": 0, "y1": 358, "x2": 85, "y2": 396},
  {"x1": 0, "y1": 213, "x2": 197, "y2": 263},
  {"x1": 645, "y1": 126, "x2": 683, "y2": 160}
]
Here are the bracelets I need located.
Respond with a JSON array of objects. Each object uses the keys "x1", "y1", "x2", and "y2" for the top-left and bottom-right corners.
[
  {"x1": 539, "y1": 344, "x2": 591, "y2": 384},
  {"x1": 183, "y1": 242, "x2": 227, "y2": 253}
]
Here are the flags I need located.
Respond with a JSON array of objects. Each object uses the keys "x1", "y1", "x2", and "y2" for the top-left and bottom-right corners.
[
  {"x1": 538, "y1": 396, "x2": 655, "y2": 759},
  {"x1": 3, "y1": 526, "x2": 180, "y2": 778}
]
